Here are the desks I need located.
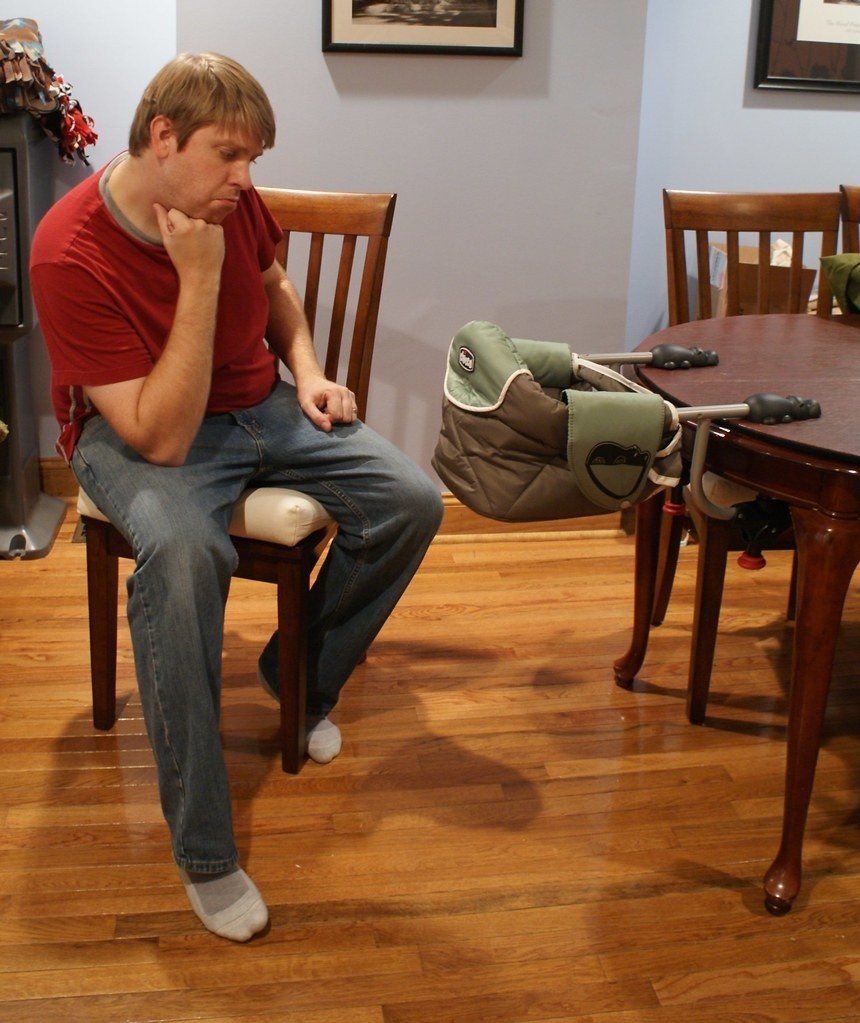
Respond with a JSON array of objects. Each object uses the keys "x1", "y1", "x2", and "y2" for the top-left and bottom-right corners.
[{"x1": 610, "y1": 315, "x2": 860, "y2": 917}]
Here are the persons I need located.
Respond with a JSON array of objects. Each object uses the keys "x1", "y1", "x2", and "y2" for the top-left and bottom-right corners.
[{"x1": 28, "y1": 49, "x2": 443, "y2": 943}]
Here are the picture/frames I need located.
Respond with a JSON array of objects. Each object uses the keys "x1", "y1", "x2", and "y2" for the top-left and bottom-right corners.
[
  {"x1": 752, "y1": 0, "x2": 860, "y2": 97},
  {"x1": 322, "y1": 0, "x2": 525, "y2": 57}
]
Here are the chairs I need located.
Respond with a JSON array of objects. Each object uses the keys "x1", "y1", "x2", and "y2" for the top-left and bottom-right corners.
[
  {"x1": 75, "y1": 190, "x2": 399, "y2": 774},
  {"x1": 650, "y1": 182, "x2": 860, "y2": 724}
]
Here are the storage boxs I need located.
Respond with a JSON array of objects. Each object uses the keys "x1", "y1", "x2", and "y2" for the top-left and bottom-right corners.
[{"x1": 693, "y1": 241, "x2": 818, "y2": 321}]
